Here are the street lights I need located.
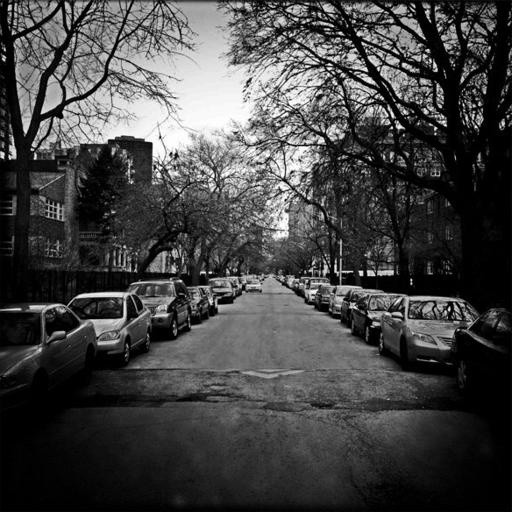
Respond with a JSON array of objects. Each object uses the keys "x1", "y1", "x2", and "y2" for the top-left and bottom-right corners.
[
  {"x1": 284, "y1": 209, "x2": 343, "y2": 287},
  {"x1": 324, "y1": 185, "x2": 345, "y2": 284}
]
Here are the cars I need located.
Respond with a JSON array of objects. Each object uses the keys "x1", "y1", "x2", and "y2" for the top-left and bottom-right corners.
[
  {"x1": 0, "y1": 301, "x2": 96, "y2": 421},
  {"x1": 183, "y1": 274, "x2": 384, "y2": 327},
  {"x1": 450, "y1": 306, "x2": 511, "y2": 410},
  {"x1": 350, "y1": 289, "x2": 417, "y2": 345},
  {"x1": 63, "y1": 292, "x2": 153, "y2": 369},
  {"x1": 377, "y1": 295, "x2": 480, "y2": 371}
]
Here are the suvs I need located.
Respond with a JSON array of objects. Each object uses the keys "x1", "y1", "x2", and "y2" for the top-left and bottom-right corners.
[{"x1": 123, "y1": 277, "x2": 194, "y2": 338}]
